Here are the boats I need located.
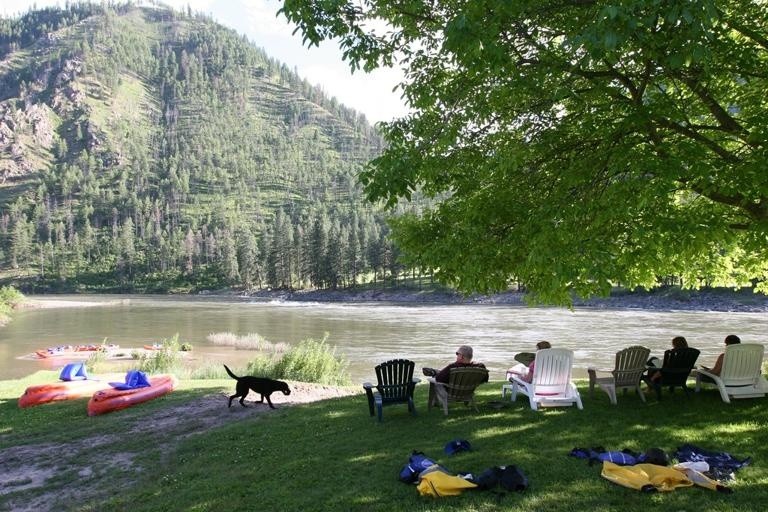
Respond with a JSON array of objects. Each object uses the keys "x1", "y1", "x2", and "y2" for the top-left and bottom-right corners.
[
  {"x1": 34, "y1": 344, "x2": 119, "y2": 358},
  {"x1": 86, "y1": 374, "x2": 173, "y2": 416},
  {"x1": 18, "y1": 372, "x2": 128, "y2": 409},
  {"x1": 144, "y1": 344, "x2": 162, "y2": 350}
]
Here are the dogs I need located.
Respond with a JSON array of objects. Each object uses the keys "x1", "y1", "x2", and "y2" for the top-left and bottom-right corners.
[{"x1": 222, "y1": 362, "x2": 291, "y2": 411}]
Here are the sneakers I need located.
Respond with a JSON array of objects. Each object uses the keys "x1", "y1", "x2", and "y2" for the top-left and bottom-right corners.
[{"x1": 422, "y1": 368, "x2": 436, "y2": 377}]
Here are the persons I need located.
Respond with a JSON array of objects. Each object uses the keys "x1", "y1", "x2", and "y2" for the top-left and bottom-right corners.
[
  {"x1": 688, "y1": 335, "x2": 743, "y2": 377},
  {"x1": 642, "y1": 336, "x2": 688, "y2": 394},
  {"x1": 421, "y1": 344, "x2": 487, "y2": 388},
  {"x1": 519, "y1": 342, "x2": 552, "y2": 383}
]
